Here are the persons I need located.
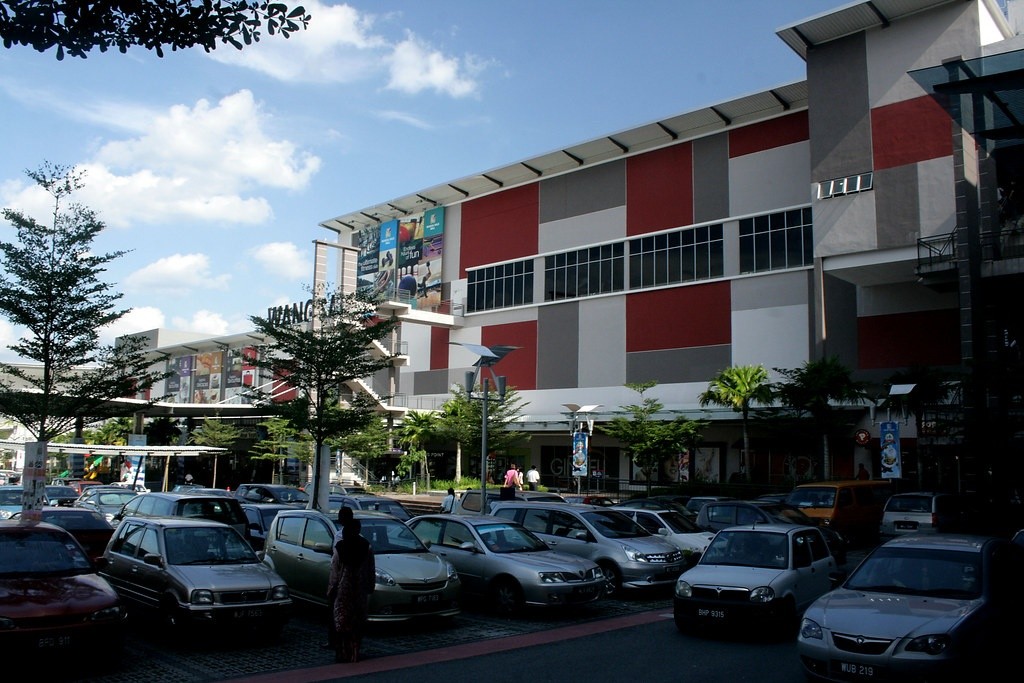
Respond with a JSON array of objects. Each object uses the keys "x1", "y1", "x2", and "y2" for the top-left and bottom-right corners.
[
  {"x1": 855, "y1": 463, "x2": 870, "y2": 481},
  {"x1": 525, "y1": 464, "x2": 540, "y2": 491},
  {"x1": 460, "y1": 487, "x2": 472, "y2": 497},
  {"x1": 333, "y1": 518, "x2": 376, "y2": 663},
  {"x1": 319, "y1": 507, "x2": 367, "y2": 647},
  {"x1": 504, "y1": 463, "x2": 524, "y2": 490},
  {"x1": 439, "y1": 487, "x2": 461, "y2": 514},
  {"x1": 418, "y1": 266, "x2": 431, "y2": 297}
]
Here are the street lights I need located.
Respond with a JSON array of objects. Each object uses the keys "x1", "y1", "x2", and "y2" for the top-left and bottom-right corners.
[{"x1": 460, "y1": 341, "x2": 506, "y2": 514}]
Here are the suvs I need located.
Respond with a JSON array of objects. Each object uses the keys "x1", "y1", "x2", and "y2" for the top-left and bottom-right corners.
[
  {"x1": 113, "y1": 492, "x2": 252, "y2": 550},
  {"x1": 233, "y1": 484, "x2": 313, "y2": 509}
]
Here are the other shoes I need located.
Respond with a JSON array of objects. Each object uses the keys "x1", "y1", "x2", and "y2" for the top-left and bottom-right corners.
[{"x1": 322, "y1": 642, "x2": 332, "y2": 649}]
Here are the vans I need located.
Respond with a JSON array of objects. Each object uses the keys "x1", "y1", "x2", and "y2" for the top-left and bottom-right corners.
[{"x1": 788, "y1": 479, "x2": 897, "y2": 537}]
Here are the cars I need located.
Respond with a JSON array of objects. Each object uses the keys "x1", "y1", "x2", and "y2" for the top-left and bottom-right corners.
[
  {"x1": 609, "y1": 491, "x2": 789, "y2": 556},
  {"x1": 486, "y1": 498, "x2": 687, "y2": 591},
  {"x1": 883, "y1": 491, "x2": 965, "y2": 536},
  {"x1": 330, "y1": 494, "x2": 417, "y2": 525},
  {"x1": 673, "y1": 524, "x2": 850, "y2": 635},
  {"x1": 1, "y1": 485, "x2": 51, "y2": 520},
  {"x1": 798, "y1": 533, "x2": 1015, "y2": 683},
  {"x1": 172, "y1": 483, "x2": 234, "y2": 501},
  {"x1": 694, "y1": 496, "x2": 848, "y2": 564},
  {"x1": 49, "y1": 476, "x2": 151, "y2": 516},
  {"x1": 561, "y1": 494, "x2": 617, "y2": 508},
  {"x1": 42, "y1": 505, "x2": 118, "y2": 568},
  {"x1": 98, "y1": 517, "x2": 294, "y2": 631},
  {"x1": 387, "y1": 513, "x2": 606, "y2": 608},
  {"x1": 458, "y1": 488, "x2": 570, "y2": 521},
  {"x1": 44, "y1": 485, "x2": 81, "y2": 507},
  {"x1": 261, "y1": 508, "x2": 462, "y2": 619},
  {"x1": 0, "y1": 469, "x2": 22, "y2": 485},
  {"x1": 1, "y1": 519, "x2": 130, "y2": 662},
  {"x1": 304, "y1": 482, "x2": 368, "y2": 496}
]
